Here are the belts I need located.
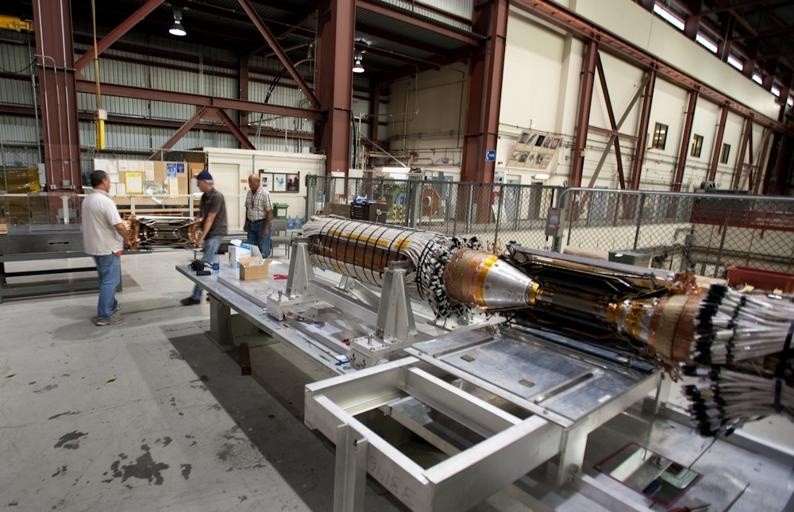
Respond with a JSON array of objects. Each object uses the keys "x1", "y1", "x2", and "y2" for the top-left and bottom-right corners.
[{"x1": 247, "y1": 217, "x2": 267, "y2": 224}]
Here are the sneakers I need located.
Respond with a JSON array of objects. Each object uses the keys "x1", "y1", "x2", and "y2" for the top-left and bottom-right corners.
[
  {"x1": 107, "y1": 303, "x2": 123, "y2": 316},
  {"x1": 180, "y1": 297, "x2": 201, "y2": 306},
  {"x1": 96, "y1": 315, "x2": 124, "y2": 326}
]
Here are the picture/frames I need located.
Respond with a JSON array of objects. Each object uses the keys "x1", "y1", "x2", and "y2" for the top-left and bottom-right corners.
[{"x1": 258, "y1": 170, "x2": 301, "y2": 193}]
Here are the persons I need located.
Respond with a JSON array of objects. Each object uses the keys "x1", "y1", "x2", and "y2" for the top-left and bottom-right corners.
[
  {"x1": 179, "y1": 170, "x2": 228, "y2": 305},
  {"x1": 80, "y1": 170, "x2": 130, "y2": 325},
  {"x1": 242, "y1": 173, "x2": 275, "y2": 260}
]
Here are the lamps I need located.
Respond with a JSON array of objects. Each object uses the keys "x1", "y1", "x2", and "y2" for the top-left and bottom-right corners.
[
  {"x1": 351, "y1": 50, "x2": 365, "y2": 74},
  {"x1": 167, "y1": 5, "x2": 190, "y2": 38}
]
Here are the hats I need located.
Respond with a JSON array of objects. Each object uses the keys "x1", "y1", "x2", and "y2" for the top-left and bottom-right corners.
[{"x1": 194, "y1": 169, "x2": 213, "y2": 180}]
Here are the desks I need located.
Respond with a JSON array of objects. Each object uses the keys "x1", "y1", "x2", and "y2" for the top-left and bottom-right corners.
[{"x1": 173, "y1": 262, "x2": 435, "y2": 378}]
